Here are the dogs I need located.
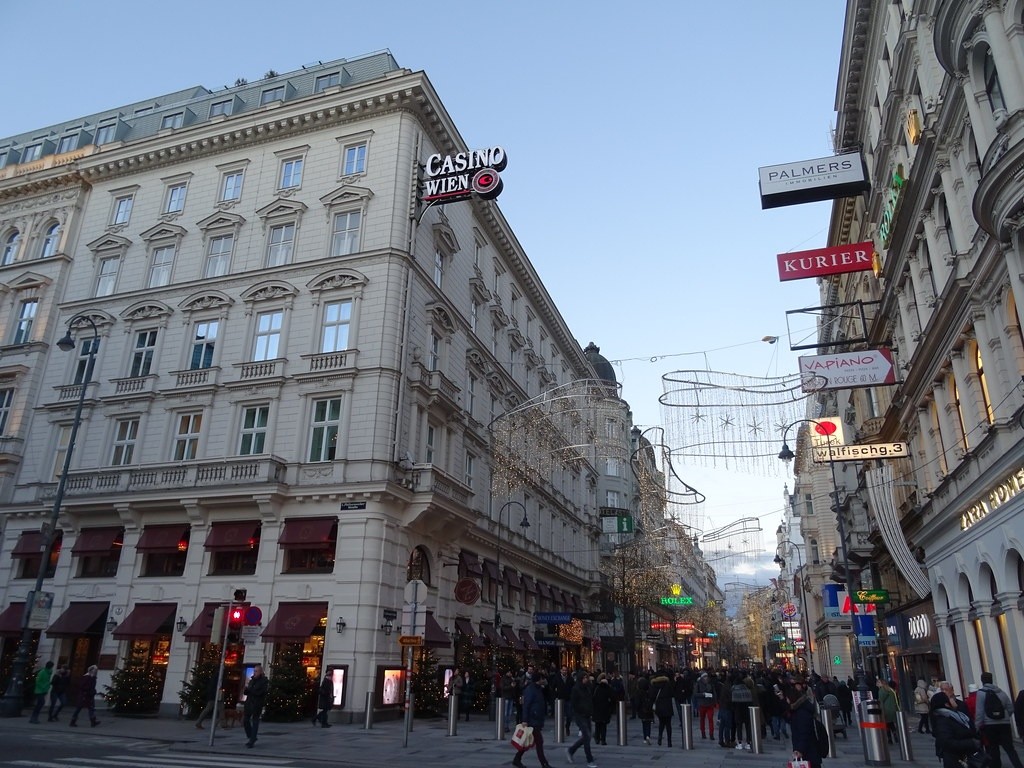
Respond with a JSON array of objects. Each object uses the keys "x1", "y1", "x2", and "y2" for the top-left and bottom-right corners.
[{"x1": 216, "y1": 709, "x2": 243, "y2": 729}]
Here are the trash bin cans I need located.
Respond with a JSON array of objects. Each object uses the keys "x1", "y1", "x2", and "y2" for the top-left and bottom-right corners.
[{"x1": 857, "y1": 699, "x2": 891, "y2": 767}]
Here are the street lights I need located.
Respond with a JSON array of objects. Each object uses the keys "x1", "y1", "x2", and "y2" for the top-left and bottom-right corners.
[
  {"x1": 771, "y1": 588, "x2": 798, "y2": 672},
  {"x1": 773, "y1": 541, "x2": 817, "y2": 688},
  {"x1": 778, "y1": 420, "x2": 869, "y2": 702},
  {"x1": 0, "y1": 316, "x2": 98, "y2": 716},
  {"x1": 489, "y1": 501, "x2": 530, "y2": 721}
]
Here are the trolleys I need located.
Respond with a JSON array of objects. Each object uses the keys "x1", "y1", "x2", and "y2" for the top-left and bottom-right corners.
[{"x1": 818, "y1": 694, "x2": 847, "y2": 737}]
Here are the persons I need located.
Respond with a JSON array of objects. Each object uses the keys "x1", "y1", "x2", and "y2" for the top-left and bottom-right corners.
[
  {"x1": 443, "y1": 660, "x2": 901, "y2": 768},
  {"x1": 28, "y1": 661, "x2": 55, "y2": 722},
  {"x1": 914, "y1": 674, "x2": 1024, "y2": 768},
  {"x1": 241, "y1": 665, "x2": 268, "y2": 750},
  {"x1": 48, "y1": 663, "x2": 72, "y2": 721},
  {"x1": 68, "y1": 663, "x2": 101, "y2": 728},
  {"x1": 194, "y1": 665, "x2": 231, "y2": 729},
  {"x1": 306, "y1": 670, "x2": 336, "y2": 729}
]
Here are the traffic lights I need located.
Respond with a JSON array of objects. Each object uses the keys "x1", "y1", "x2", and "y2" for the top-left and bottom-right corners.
[
  {"x1": 233, "y1": 590, "x2": 246, "y2": 600},
  {"x1": 233, "y1": 611, "x2": 240, "y2": 619}
]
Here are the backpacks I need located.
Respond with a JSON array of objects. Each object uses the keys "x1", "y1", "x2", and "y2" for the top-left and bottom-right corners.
[{"x1": 978, "y1": 688, "x2": 1005, "y2": 721}]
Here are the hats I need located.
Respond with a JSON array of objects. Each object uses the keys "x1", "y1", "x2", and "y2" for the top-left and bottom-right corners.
[
  {"x1": 968, "y1": 683, "x2": 979, "y2": 693},
  {"x1": 700, "y1": 670, "x2": 708, "y2": 678}
]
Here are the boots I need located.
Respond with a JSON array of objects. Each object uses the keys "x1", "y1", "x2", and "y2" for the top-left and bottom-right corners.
[
  {"x1": 29, "y1": 709, "x2": 41, "y2": 724},
  {"x1": 69, "y1": 718, "x2": 78, "y2": 727},
  {"x1": 90, "y1": 718, "x2": 101, "y2": 727}
]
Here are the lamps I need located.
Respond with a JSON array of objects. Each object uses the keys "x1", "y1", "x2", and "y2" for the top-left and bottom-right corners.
[
  {"x1": 106, "y1": 616, "x2": 117, "y2": 631},
  {"x1": 177, "y1": 616, "x2": 187, "y2": 632},
  {"x1": 335, "y1": 616, "x2": 346, "y2": 633},
  {"x1": 381, "y1": 618, "x2": 393, "y2": 636},
  {"x1": 444, "y1": 626, "x2": 450, "y2": 638}
]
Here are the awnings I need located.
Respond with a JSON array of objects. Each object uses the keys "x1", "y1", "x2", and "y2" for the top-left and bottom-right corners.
[
  {"x1": 134, "y1": 525, "x2": 189, "y2": 553},
  {"x1": 70, "y1": 527, "x2": 123, "y2": 557},
  {"x1": 276, "y1": 516, "x2": 337, "y2": 551},
  {"x1": 11, "y1": 529, "x2": 63, "y2": 560},
  {"x1": 460, "y1": 551, "x2": 583, "y2": 611},
  {"x1": 182, "y1": 603, "x2": 241, "y2": 643},
  {"x1": 0, "y1": 603, "x2": 41, "y2": 638},
  {"x1": 111, "y1": 602, "x2": 177, "y2": 641},
  {"x1": 259, "y1": 602, "x2": 329, "y2": 644},
  {"x1": 203, "y1": 519, "x2": 262, "y2": 553},
  {"x1": 423, "y1": 609, "x2": 543, "y2": 653},
  {"x1": 45, "y1": 601, "x2": 109, "y2": 641}
]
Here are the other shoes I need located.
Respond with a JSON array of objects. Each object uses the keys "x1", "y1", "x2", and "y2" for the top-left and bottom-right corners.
[
  {"x1": 542, "y1": 765, "x2": 554, "y2": 768},
  {"x1": 565, "y1": 748, "x2": 574, "y2": 763},
  {"x1": 512, "y1": 758, "x2": 527, "y2": 768},
  {"x1": 925, "y1": 730, "x2": 932, "y2": 734},
  {"x1": 195, "y1": 724, "x2": 205, "y2": 730},
  {"x1": 48, "y1": 717, "x2": 56, "y2": 722},
  {"x1": 246, "y1": 738, "x2": 258, "y2": 747},
  {"x1": 587, "y1": 762, "x2": 597, "y2": 768},
  {"x1": 702, "y1": 730, "x2": 789, "y2": 750},
  {"x1": 53, "y1": 716, "x2": 59, "y2": 721},
  {"x1": 310, "y1": 718, "x2": 332, "y2": 728},
  {"x1": 566, "y1": 726, "x2": 672, "y2": 747},
  {"x1": 918, "y1": 729, "x2": 925, "y2": 734}
]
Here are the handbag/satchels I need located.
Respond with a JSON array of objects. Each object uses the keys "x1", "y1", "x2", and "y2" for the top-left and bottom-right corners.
[
  {"x1": 511, "y1": 724, "x2": 534, "y2": 752},
  {"x1": 788, "y1": 756, "x2": 810, "y2": 768},
  {"x1": 962, "y1": 748, "x2": 993, "y2": 768},
  {"x1": 812, "y1": 716, "x2": 830, "y2": 759},
  {"x1": 914, "y1": 690, "x2": 926, "y2": 704},
  {"x1": 652, "y1": 703, "x2": 656, "y2": 710}
]
